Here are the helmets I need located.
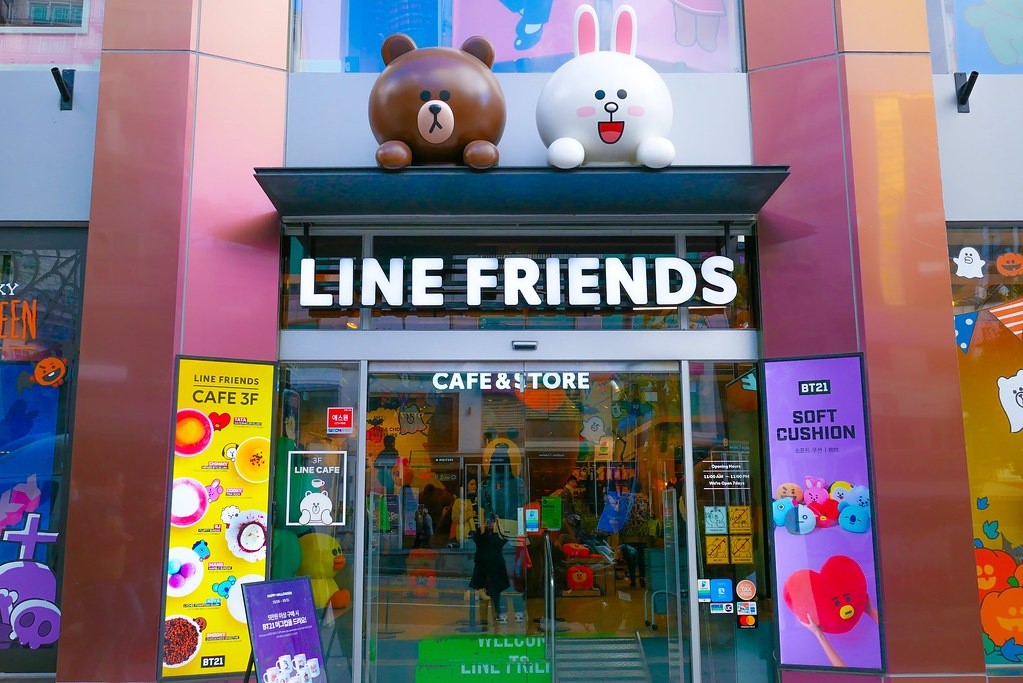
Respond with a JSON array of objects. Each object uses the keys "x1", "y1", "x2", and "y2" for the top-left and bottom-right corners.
[{"x1": 567, "y1": 514, "x2": 581, "y2": 521}]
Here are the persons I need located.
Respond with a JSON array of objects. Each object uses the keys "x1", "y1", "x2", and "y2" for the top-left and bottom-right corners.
[
  {"x1": 618, "y1": 496, "x2": 656, "y2": 589},
  {"x1": 468, "y1": 512, "x2": 512, "y2": 636},
  {"x1": 422, "y1": 508, "x2": 433, "y2": 549},
  {"x1": 564, "y1": 475, "x2": 584, "y2": 542},
  {"x1": 451, "y1": 478, "x2": 492, "y2": 600},
  {"x1": 414, "y1": 510, "x2": 423, "y2": 548}
]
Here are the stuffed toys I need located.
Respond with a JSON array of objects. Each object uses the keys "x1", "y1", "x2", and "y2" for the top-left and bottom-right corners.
[
  {"x1": 773, "y1": 475, "x2": 871, "y2": 535},
  {"x1": 269, "y1": 437, "x2": 326, "y2": 579},
  {"x1": 296, "y1": 532, "x2": 351, "y2": 608},
  {"x1": 783, "y1": 554, "x2": 868, "y2": 634}
]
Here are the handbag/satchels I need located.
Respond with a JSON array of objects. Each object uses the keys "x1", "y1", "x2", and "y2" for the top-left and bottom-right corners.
[
  {"x1": 647, "y1": 517, "x2": 658, "y2": 536},
  {"x1": 493, "y1": 518, "x2": 530, "y2": 546},
  {"x1": 434, "y1": 502, "x2": 454, "y2": 533}
]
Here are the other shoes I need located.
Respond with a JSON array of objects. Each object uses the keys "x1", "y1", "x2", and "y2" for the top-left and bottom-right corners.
[
  {"x1": 639, "y1": 576, "x2": 645, "y2": 588},
  {"x1": 476, "y1": 588, "x2": 491, "y2": 600},
  {"x1": 630, "y1": 576, "x2": 636, "y2": 587},
  {"x1": 496, "y1": 614, "x2": 507, "y2": 623},
  {"x1": 514, "y1": 612, "x2": 526, "y2": 622}
]
